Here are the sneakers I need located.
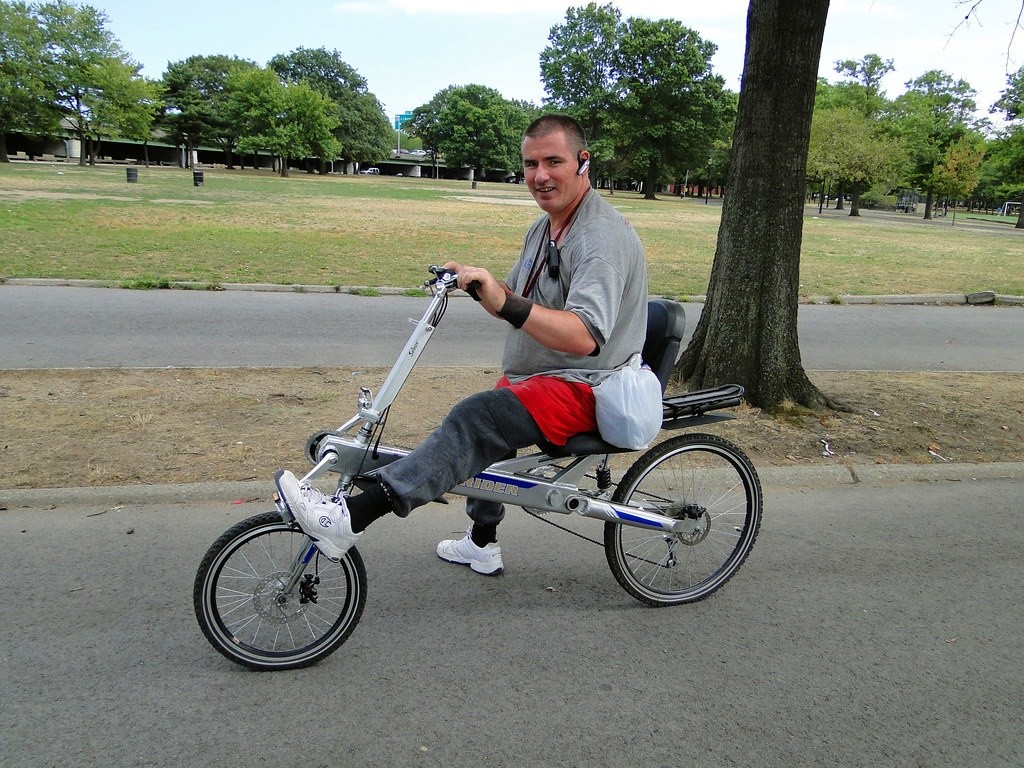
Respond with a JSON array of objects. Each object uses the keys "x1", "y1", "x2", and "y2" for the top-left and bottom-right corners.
[
  {"x1": 274, "y1": 469, "x2": 365, "y2": 563},
  {"x1": 436, "y1": 526, "x2": 504, "y2": 574}
]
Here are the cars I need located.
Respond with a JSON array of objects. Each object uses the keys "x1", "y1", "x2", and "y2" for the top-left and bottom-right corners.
[{"x1": 392, "y1": 148, "x2": 430, "y2": 155}]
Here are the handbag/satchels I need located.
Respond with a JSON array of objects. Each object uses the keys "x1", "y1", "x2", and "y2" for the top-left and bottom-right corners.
[{"x1": 591, "y1": 354, "x2": 663, "y2": 451}]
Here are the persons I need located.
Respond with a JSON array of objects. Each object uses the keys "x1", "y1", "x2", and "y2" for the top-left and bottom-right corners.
[{"x1": 275, "y1": 115, "x2": 648, "y2": 575}]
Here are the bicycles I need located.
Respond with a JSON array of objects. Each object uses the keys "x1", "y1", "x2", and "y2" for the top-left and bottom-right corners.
[{"x1": 193, "y1": 265, "x2": 766, "y2": 672}]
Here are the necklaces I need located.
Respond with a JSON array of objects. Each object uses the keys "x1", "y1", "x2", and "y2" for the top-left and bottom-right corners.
[{"x1": 521, "y1": 186, "x2": 592, "y2": 299}]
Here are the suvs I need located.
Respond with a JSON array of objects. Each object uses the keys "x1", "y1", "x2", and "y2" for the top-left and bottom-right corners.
[{"x1": 360, "y1": 167, "x2": 380, "y2": 174}]
